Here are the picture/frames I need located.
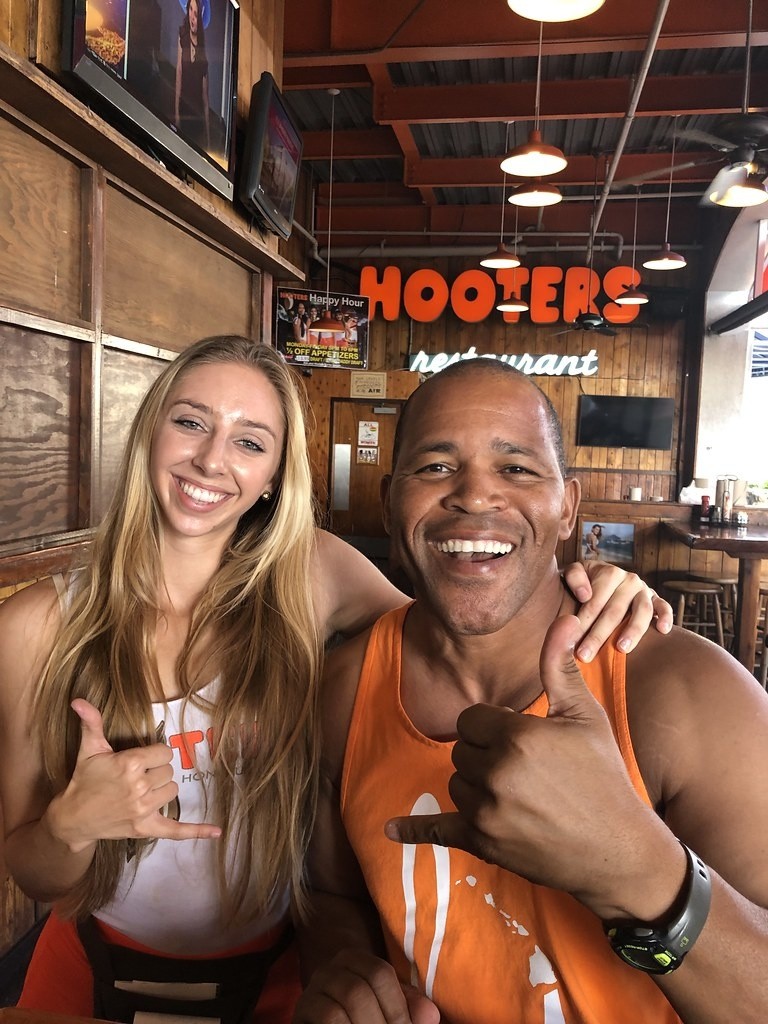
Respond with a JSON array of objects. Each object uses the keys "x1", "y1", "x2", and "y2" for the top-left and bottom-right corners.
[{"x1": 576, "y1": 515, "x2": 645, "y2": 573}]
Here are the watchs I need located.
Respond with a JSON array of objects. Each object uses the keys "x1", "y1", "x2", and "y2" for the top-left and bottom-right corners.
[{"x1": 603, "y1": 841, "x2": 711, "y2": 974}]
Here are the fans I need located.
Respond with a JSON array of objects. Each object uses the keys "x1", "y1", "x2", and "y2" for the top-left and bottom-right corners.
[
  {"x1": 608, "y1": 0, "x2": 767, "y2": 191},
  {"x1": 548, "y1": 147, "x2": 633, "y2": 339}
]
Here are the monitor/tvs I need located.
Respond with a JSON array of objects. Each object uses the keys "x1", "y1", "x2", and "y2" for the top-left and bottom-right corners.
[
  {"x1": 575, "y1": 394, "x2": 674, "y2": 451},
  {"x1": 237, "y1": 71, "x2": 304, "y2": 241},
  {"x1": 59, "y1": 0, "x2": 238, "y2": 203}
]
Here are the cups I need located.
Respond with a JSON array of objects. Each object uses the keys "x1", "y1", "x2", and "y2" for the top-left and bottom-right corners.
[
  {"x1": 631, "y1": 488, "x2": 642, "y2": 501},
  {"x1": 650, "y1": 497, "x2": 663, "y2": 502}
]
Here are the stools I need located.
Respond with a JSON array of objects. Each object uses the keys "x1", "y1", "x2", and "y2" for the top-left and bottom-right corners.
[
  {"x1": 684, "y1": 571, "x2": 739, "y2": 642},
  {"x1": 752, "y1": 581, "x2": 768, "y2": 691},
  {"x1": 661, "y1": 581, "x2": 724, "y2": 652}
]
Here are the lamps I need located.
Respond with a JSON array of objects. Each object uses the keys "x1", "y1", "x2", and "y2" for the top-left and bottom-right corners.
[
  {"x1": 305, "y1": 85, "x2": 348, "y2": 331},
  {"x1": 480, "y1": 1, "x2": 767, "y2": 311}
]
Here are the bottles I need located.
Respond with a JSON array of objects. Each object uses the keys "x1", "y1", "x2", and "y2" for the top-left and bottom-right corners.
[
  {"x1": 721, "y1": 480, "x2": 731, "y2": 527},
  {"x1": 699, "y1": 496, "x2": 710, "y2": 526},
  {"x1": 712, "y1": 507, "x2": 721, "y2": 524}
]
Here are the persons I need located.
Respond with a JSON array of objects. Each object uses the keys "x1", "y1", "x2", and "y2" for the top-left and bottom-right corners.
[
  {"x1": 0, "y1": 335, "x2": 673, "y2": 1024},
  {"x1": 293, "y1": 303, "x2": 352, "y2": 344},
  {"x1": 175, "y1": 0, "x2": 212, "y2": 154},
  {"x1": 292, "y1": 357, "x2": 768, "y2": 1024},
  {"x1": 585, "y1": 524, "x2": 602, "y2": 560}
]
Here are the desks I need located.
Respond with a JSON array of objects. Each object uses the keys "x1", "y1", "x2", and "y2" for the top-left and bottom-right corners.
[{"x1": 662, "y1": 520, "x2": 768, "y2": 673}]
